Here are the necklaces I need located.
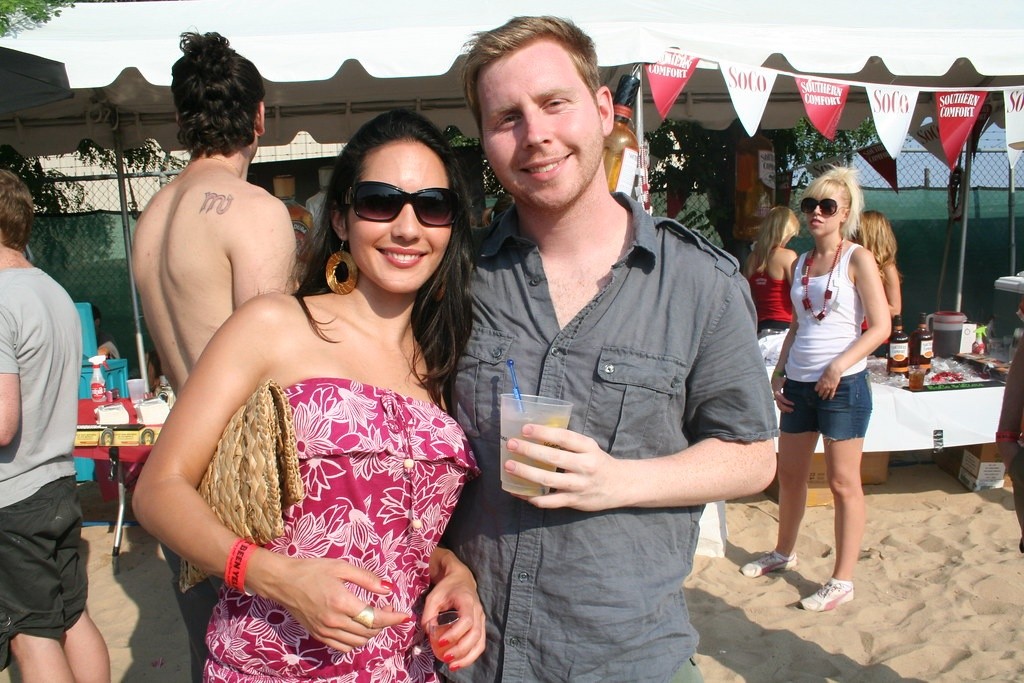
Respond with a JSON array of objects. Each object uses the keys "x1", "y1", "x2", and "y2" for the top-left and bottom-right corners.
[{"x1": 801, "y1": 239, "x2": 842, "y2": 321}]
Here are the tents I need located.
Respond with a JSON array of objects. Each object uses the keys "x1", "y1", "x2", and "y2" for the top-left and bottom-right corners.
[{"x1": 0, "y1": 0, "x2": 1024, "y2": 312}]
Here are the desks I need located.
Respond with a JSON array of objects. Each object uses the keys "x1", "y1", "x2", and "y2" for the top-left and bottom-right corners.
[{"x1": 72, "y1": 340, "x2": 1019, "y2": 575}]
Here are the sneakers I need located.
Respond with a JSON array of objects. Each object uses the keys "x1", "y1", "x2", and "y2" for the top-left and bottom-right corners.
[
  {"x1": 740, "y1": 550, "x2": 797, "y2": 578},
  {"x1": 798, "y1": 579, "x2": 853, "y2": 612}
]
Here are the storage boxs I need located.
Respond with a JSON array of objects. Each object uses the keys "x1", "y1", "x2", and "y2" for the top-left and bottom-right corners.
[
  {"x1": 860, "y1": 451, "x2": 890, "y2": 484},
  {"x1": 994, "y1": 271, "x2": 1024, "y2": 343},
  {"x1": 762, "y1": 453, "x2": 833, "y2": 507},
  {"x1": 931, "y1": 442, "x2": 1007, "y2": 492}
]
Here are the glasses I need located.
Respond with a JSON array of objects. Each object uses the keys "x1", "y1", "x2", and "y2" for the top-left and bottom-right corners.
[
  {"x1": 801, "y1": 197, "x2": 837, "y2": 215},
  {"x1": 343, "y1": 180, "x2": 460, "y2": 227}
]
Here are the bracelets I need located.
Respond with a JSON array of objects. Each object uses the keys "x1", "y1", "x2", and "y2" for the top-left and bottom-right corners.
[
  {"x1": 224, "y1": 538, "x2": 259, "y2": 596},
  {"x1": 771, "y1": 370, "x2": 785, "y2": 377}
]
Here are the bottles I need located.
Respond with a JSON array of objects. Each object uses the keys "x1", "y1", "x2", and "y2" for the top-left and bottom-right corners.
[
  {"x1": 909, "y1": 313, "x2": 933, "y2": 374},
  {"x1": 886, "y1": 315, "x2": 910, "y2": 379},
  {"x1": 272, "y1": 174, "x2": 314, "y2": 284},
  {"x1": 306, "y1": 165, "x2": 335, "y2": 256}
]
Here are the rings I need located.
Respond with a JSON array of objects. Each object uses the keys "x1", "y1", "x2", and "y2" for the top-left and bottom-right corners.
[{"x1": 352, "y1": 605, "x2": 374, "y2": 629}]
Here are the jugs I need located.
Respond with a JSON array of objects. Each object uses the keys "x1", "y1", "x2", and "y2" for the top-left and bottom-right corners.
[{"x1": 926, "y1": 311, "x2": 967, "y2": 358}]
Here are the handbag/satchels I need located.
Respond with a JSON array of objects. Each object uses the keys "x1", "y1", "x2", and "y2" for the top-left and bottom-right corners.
[{"x1": 178, "y1": 380, "x2": 304, "y2": 594}]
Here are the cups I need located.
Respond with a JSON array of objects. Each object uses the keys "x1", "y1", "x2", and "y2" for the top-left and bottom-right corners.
[
  {"x1": 499, "y1": 392, "x2": 572, "y2": 497},
  {"x1": 427, "y1": 611, "x2": 462, "y2": 663},
  {"x1": 126, "y1": 378, "x2": 146, "y2": 403},
  {"x1": 909, "y1": 366, "x2": 926, "y2": 391},
  {"x1": 761, "y1": 328, "x2": 784, "y2": 339}
]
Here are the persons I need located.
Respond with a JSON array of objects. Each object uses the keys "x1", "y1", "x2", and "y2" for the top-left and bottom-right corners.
[
  {"x1": 996, "y1": 300, "x2": 1024, "y2": 554},
  {"x1": 745, "y1": 206, "x2": 800, "y2": 334},
  {"x1": 741, "y1": 163, "x2": 891, "y2": 612},
  {"x1": 851, "y1": 210, "x2": 903, "y2": 357},
  {"x1": 133, "y1": 31, "x2": 296, "y2": 683},
  {"x1": 436, "y1": 16, "x2": 776, "y2": 683},
  {"x1": 131, "y1": 111, "x2": 486, "y2": 683},
  {"x1": 0, "y1": 170, "x2": 111, "y2": 683},
  {"x1": 92, "y1": 304, "x2": 161, "y2": 397}
]
